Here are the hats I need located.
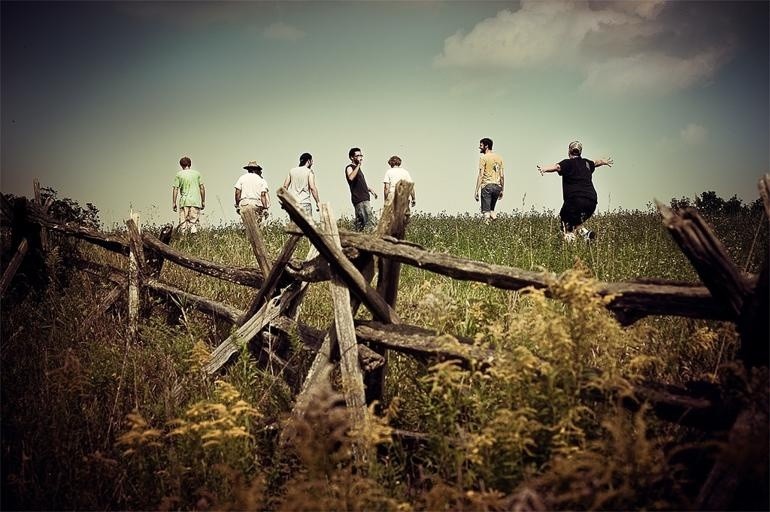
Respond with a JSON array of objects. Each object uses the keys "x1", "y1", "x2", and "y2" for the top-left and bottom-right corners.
[
  {"x1": 299, "y1": 153, "x2": 312, "y2": 166},
  {"x1": 569, "y1": 141, "x2": 583, "y2": 155},
  {"x1": 244, "y1": 161, "x2": 262, "y2": 170},
  {"x1": 388, "y1": 156, "x2": 401, "y2": 165}
]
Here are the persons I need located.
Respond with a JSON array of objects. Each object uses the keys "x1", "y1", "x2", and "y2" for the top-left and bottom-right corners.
[
  {"x1": 474, "y1": 138, "x2": 505, "y2": 223},
  {"x1": 534, "y1": 139, "x2": 613, "y2": 245},
  {"x1": 344, "y1": 147, "x2": 378, "y2": 230},
  {"x1": 172, "y1": 156, "x2": 206, "y2": 235},
  {"x1": 230, "y1": 159, "x2": 269, "y2": 232},
  {"x1": 283, "y1": 153, "x2": 320, "y2": 219},
  {"x1": 381, "y1": 156, "x2": 416, "y2": 207}
]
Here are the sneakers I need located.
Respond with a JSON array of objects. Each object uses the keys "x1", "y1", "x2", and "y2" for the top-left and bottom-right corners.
[{"x1": 587, "y1": 231, "x2": 595, "y2": 244}]
